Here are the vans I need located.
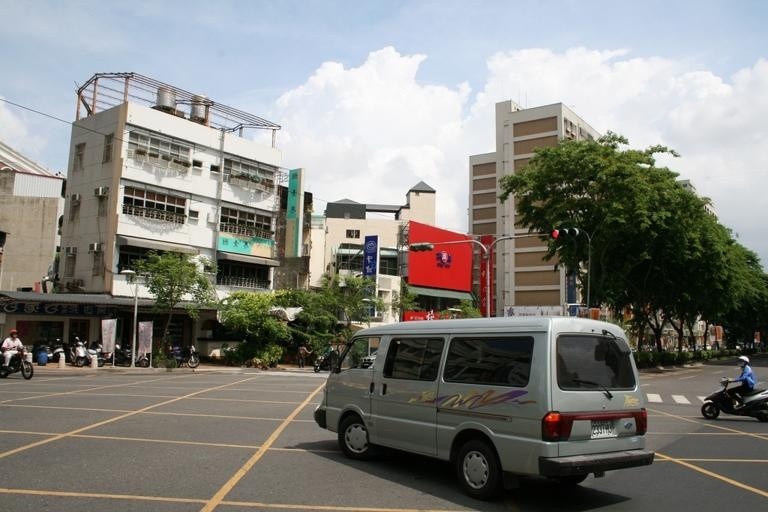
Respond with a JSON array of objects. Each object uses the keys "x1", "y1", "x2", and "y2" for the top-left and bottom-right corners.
[{"x1": 314, "y1": 315, "x2": 654, "y2": 501}]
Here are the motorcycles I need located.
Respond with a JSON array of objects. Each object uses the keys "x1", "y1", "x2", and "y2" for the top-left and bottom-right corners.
[
  {"x1": 0, "y1": 347, "x2": 34, "y2": 379},
  {"x1": 33, "y1": 336, "x2": 200, "y2": 368},
  {"x1": 701, "y1": 376, "x2": 768, "y2": 422},
  {"x1": 314, "y1": 356, "x2": 325, "y2": 373}
]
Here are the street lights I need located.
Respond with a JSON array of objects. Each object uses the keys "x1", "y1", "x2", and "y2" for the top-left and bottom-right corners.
[
  {"x1": 120, "y1": 269, "x2": 139, "y2": 368},
  {"x1": 362, "y1": 298, "x2": 372, "y2": 328}
]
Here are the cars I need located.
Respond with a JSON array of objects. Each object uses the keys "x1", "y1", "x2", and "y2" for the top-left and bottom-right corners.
[
  {"x1": 361, "y1": 350, "x2": 378, "y2": 368},
  {"x1": 641, "y1": 344, "x2": 711, "y2": 352}
]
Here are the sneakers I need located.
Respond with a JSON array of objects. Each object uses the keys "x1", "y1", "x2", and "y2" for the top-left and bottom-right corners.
[{"x1": 734, "y1": 400, "x2": 746, "y2": 409}]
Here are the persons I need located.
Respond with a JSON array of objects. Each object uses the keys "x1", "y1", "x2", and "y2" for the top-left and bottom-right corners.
[
  {"x1": 297, "y1": 342, "x2": 312, "y2": 369},
  {"x1": 0, "y1": 328, "x2": 25, "y2": 370},
  {"x1": 720, "y1": 356, "x2": 756, "y2": 410}
]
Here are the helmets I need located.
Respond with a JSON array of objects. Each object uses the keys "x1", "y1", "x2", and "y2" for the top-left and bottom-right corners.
[
  {"x1": 9, "y1": 329, "x2": 19, "y2": 337},
  {"x1": 738, "y1": 354, "x2": 750, "y2": 363}
]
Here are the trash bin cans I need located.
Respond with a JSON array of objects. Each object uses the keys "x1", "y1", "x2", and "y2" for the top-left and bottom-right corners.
[
  {"x1": 37, "y1": 352, "x2": 48, "y2": 366},
  {"x1": 91, "y1": 356, "x2": 97, "y2": 368},
  {"x1": 58, "y1": 353, "x2": 65, "y2": 368}
]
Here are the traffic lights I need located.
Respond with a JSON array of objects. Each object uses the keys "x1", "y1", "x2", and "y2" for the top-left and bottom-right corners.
[
  {"x1": 335, "y1": 325, "x2": 339, "y2": 337},
  {"x1": 436, "y1": 249, "x2": 451, "y2": 267},
  {"x1": 410, "y1": 242, "x2": 434, "y2": 251},
  {"x1": 551, "y1": 228, "x2": 579, "y2": 239}
]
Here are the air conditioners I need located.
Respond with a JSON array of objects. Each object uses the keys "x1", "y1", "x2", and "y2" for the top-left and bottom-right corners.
[
  {"x1": 88, "y1": 242, "x2": 98, "y2": 252},
  {"x1": 65, "y1": 246, "x2": 78, "y2": 255},
  {"x1": 71, "y1": 193, "x2": 80, "y2": 200},
  {"x1": 94, "y1": 187, "x2": 102, "y2": 197}
]
